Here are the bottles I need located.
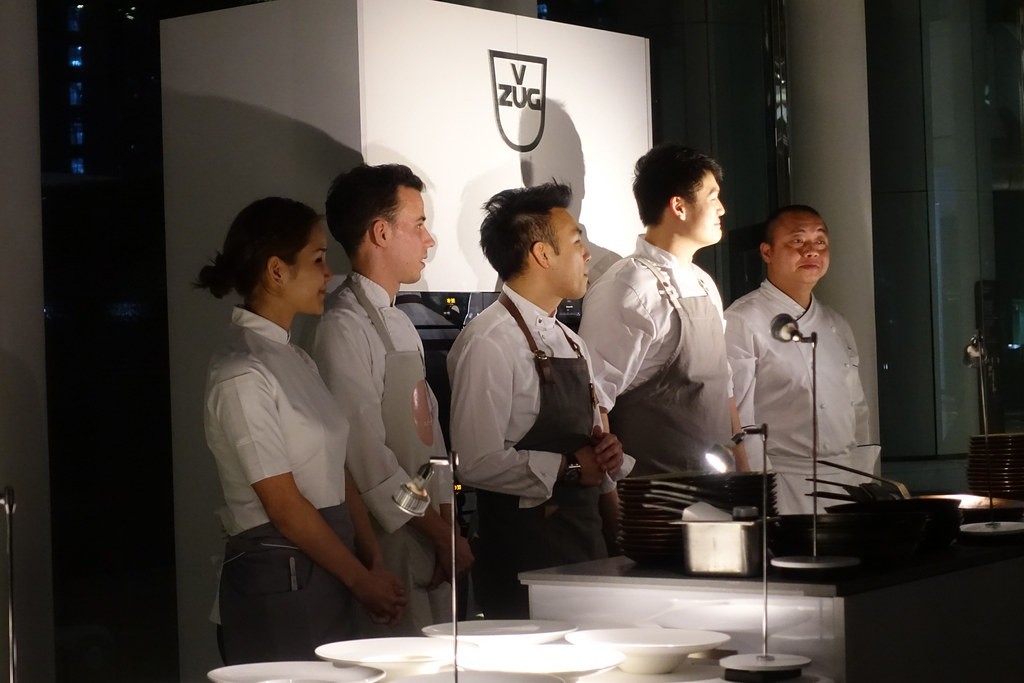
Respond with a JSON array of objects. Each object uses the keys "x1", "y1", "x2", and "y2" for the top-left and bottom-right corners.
[{"x1": 731, "y1": 505, "x2": 759, "y2": 522}]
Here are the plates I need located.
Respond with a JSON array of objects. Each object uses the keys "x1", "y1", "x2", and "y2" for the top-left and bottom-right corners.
[
  {"x1": 966, "y1": 433, "x2": 1024, "y2": 498},
  {"x1": 614, "y1": 470, "x2": 780, "y2": 563}
]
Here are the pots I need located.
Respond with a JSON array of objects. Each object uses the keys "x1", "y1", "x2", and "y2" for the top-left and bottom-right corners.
[{"x1": 765, "y1": 460, "x2": 1024, "y2": 573}]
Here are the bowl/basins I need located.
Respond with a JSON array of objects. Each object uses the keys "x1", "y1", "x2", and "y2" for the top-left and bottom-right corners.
[
  {"x1": 207, "y1": 659, "x2": 385, "y2": 683},
  {"x1": 562, "y1": 627, "x2": 733, "y2": 674},
  {"x1": 420, "y1": 620, "x2": 579, "y2": 644},
  {"x1": 314, "y1": 636, "x2": 627, "y2": 683}
]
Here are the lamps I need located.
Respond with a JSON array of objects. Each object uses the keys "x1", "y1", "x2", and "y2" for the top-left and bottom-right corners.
[
  {"x1": 392, "y1": 451, "x2": 459, "y2": 683},
  {"x1": 771, "y1": 313, "x2": 859, "y2": 569},
  {"x1": 705, "y1": 423, "x2": 811, "y2": 683},
  {"x1": 959, "y1": 331, "x2": 1024, "y2": 535}
]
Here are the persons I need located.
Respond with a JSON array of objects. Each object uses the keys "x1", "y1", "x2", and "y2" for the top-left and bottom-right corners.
[
  {"x1": 194, "y1": 196, "x2": 407, "y2": 667},
  {"x1": 579, "y1": 150, "x2": 751, "y2": 473},
  {"x1": 299, "y1": 164, "x2": 473, "y2": 638},
  {"x1": 445, "y1": 178, "x2": 636, "y2": 617},
  {"x1": 724, "y1": 204, "x2": 881, "y2": 516}
]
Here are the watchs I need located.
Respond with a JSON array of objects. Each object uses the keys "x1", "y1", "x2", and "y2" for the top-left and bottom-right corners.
[{"x1": 561, "y1": 454, "x2": 584, "y2": 488}]
